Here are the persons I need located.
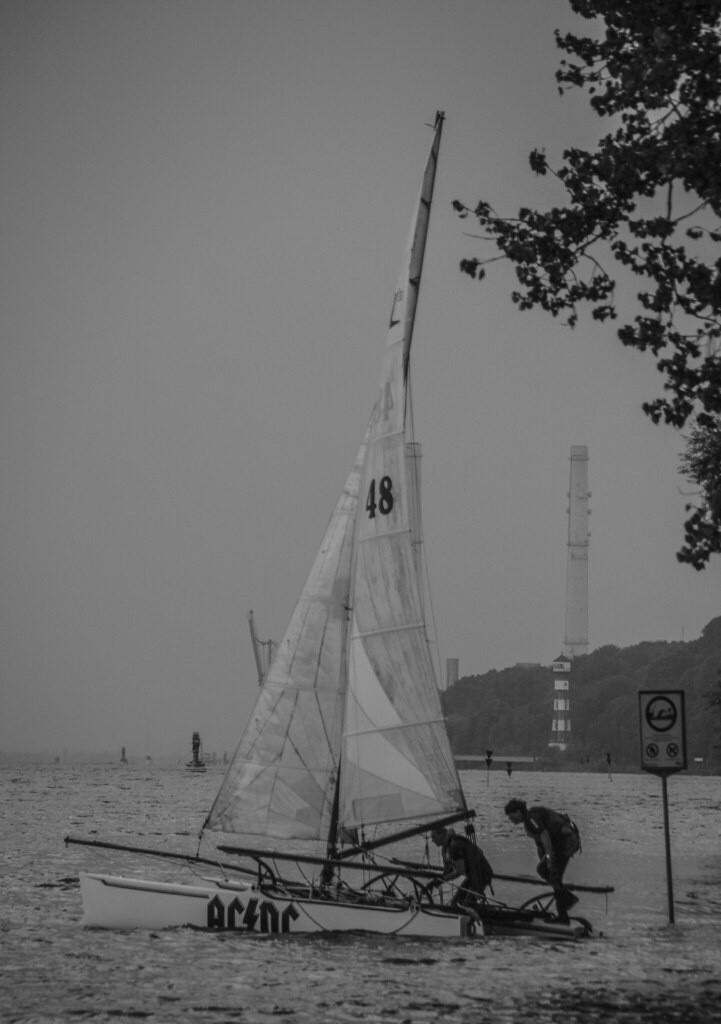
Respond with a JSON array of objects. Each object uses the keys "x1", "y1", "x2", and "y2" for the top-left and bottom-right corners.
[
  {"x1": 425, "y1": 823, "x2": 495, "y2": 904},
  {"x1": 504, "y1": 798, "x2": 581, "y2": 926}
]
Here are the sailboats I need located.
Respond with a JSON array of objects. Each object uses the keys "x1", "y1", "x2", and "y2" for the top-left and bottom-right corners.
[{"x1": 63, "y1": 109, "x2": 615, "y2": 944}]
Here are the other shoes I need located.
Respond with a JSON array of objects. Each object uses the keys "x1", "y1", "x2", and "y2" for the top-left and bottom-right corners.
[
  {"x1": 560, "y1": 888, "x2": 579, "y2": 908},
  {"x1": 544, "y1": 915, "x2": 570, "y2": 925}
]
